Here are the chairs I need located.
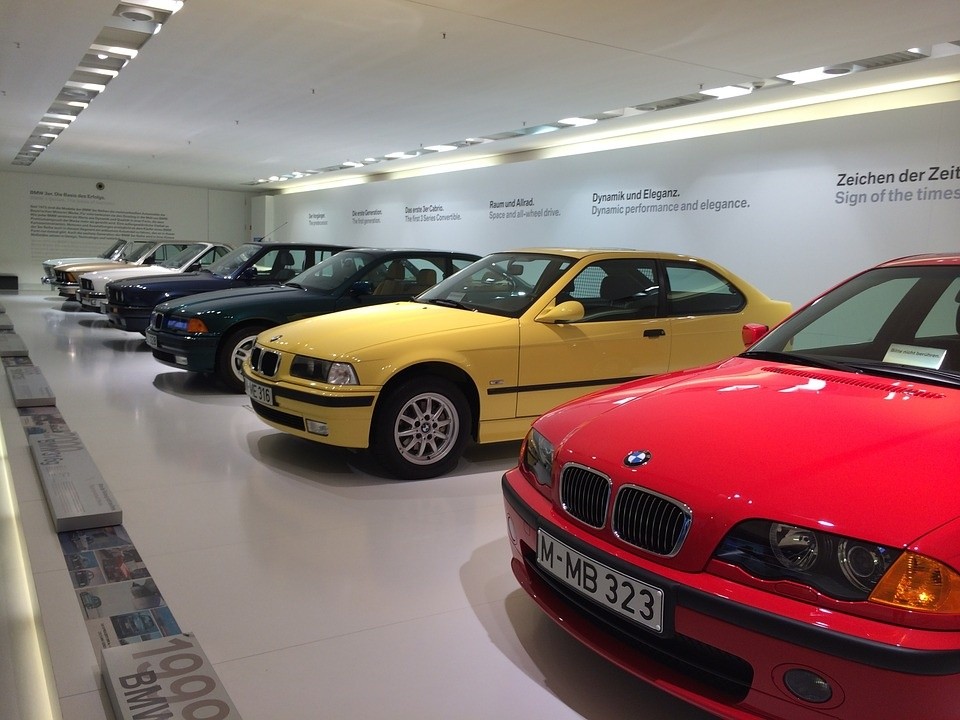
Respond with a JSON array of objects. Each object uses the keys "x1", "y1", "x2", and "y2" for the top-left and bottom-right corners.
[
  {"x1": 404, "y1": 269, "x2": 437, "y2": 294},
  {"x1": 372, "y1": 259, "x2": 405, "y2": 295},
  {"x1": 274, "y1": 253, "x2": 296, "y2": 282},
  {"x1": 586, "y1": 274, "x2": 640, "y2": 322}
]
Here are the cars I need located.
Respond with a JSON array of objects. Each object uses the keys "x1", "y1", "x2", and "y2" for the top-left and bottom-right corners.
[
  {"x1": 145, "y1": 245, "x2": 535, "y2": 394},
  {"x1": 40, "y1": 237, "x2": 273, "y2": 311},
  {"x1": 105, "y1": 243, "x2": 354, "y2": 338},
  {"x1": 240, "y1": 247, "x2": 795, "y2": 482},
  {"x1": 499, "y1": 251, "x2": 960, "y2": 720}
]
